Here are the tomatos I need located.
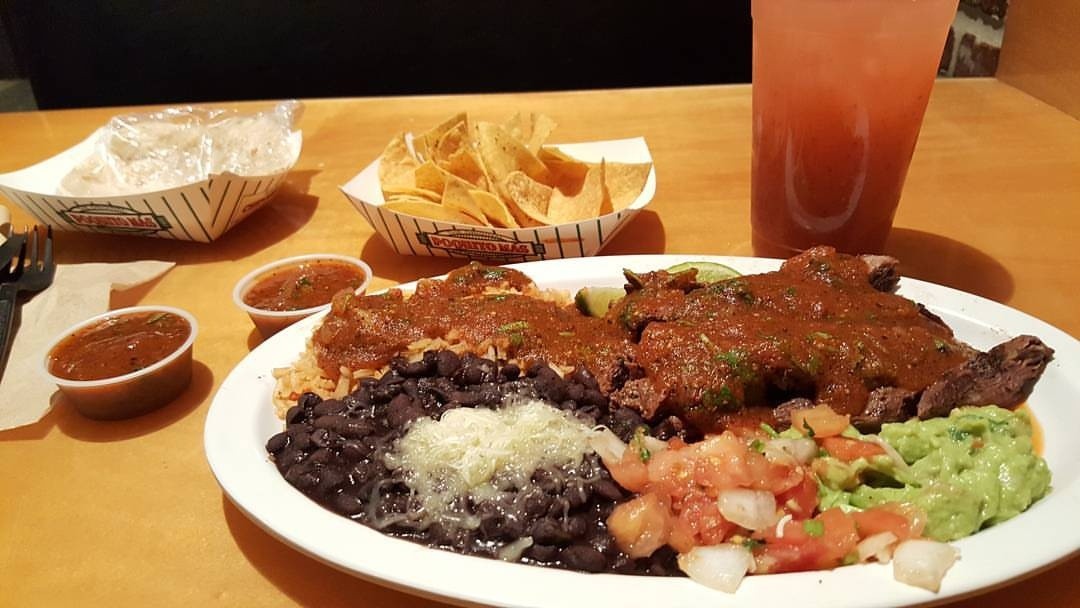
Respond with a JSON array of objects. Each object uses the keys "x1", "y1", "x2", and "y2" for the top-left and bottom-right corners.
[{"x1": 607, "y1": 401, "x2": 959, "y2": 594}]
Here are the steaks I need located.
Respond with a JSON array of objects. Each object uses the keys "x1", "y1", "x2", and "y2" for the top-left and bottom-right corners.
[{"x1": 624, "y1": 244, "x2": 1056, "y2": 428}]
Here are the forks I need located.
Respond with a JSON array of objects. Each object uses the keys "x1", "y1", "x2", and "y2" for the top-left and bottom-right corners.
[{"x1": 0, "y1": 219, "x2": 55, "y2": 381}]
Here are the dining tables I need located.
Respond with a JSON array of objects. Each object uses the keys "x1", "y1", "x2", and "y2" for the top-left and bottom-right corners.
[{"x1": 0, "y1": 79, "x2": 1079, "y2": 608}]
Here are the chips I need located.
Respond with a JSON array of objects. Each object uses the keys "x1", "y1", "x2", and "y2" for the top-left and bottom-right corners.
[{"x1": 378, "y1": 110, "x2": 653, "y2": 229}]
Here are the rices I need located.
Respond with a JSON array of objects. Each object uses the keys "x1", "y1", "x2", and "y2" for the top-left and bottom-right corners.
[{"x1": 271, "y1": 260, "x2": 589, "y2": 420}]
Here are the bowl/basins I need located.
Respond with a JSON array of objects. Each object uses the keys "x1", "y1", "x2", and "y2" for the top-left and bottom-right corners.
[
  {"x1": 236, "y1": 251, "x2": 371, "y2": 341},
  {"x1": 38, "y1": 303, "x2": 197, "y2": 423}
]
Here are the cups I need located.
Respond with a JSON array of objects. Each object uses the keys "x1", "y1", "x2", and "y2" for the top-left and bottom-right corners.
[{"x1": 749, "y1": 0, "x2": 965, "y2": 259}]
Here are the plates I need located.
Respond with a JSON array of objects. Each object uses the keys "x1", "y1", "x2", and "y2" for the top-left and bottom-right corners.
[{"x1": 200, "y1": 252, "x2": 1078, "y2": 608}]
